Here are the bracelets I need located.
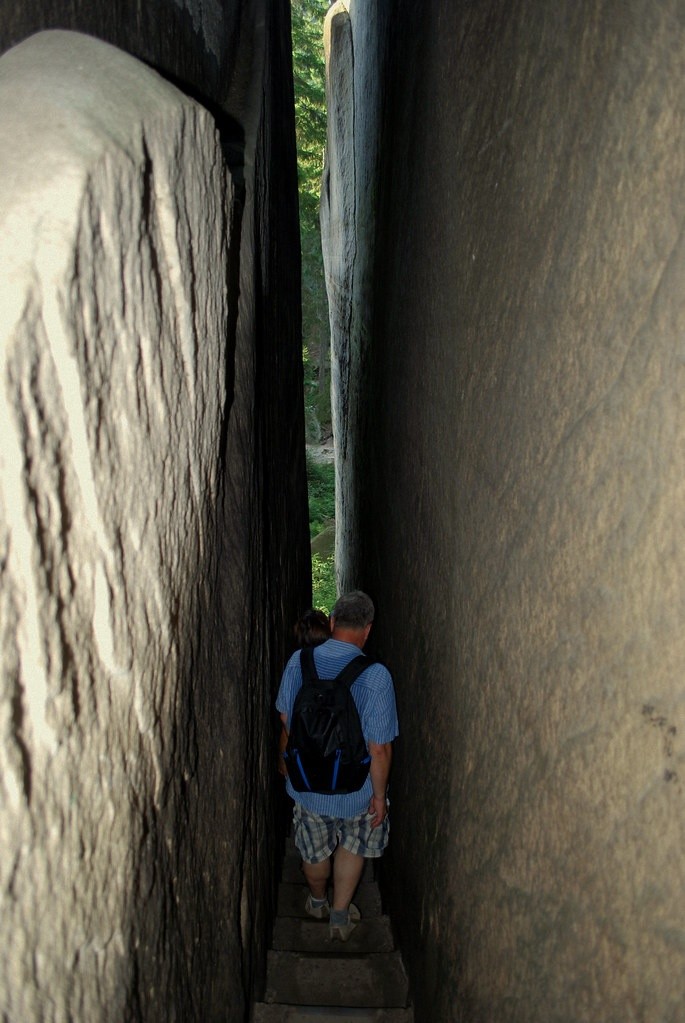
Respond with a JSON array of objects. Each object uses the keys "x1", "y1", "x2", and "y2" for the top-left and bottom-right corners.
[{"x1": 279, "y1": 753, "x2": 283, "y2": 760}]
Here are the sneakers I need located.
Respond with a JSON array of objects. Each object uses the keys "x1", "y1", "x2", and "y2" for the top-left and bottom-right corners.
[
  {"x1": 305, "y1": 893, "x2": 331, "y2": 919},
  {"x1": 330, "y1": 903, "x2": 360, "y2": 943}
]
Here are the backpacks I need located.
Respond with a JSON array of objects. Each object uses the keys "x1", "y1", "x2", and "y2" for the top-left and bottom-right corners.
[{"x1": 285, "y1": 647, "x2": 377, "y2": 793}]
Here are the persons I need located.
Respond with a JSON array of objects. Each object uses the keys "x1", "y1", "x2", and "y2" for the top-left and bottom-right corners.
[
  {"x1": 279, "y1": 610, "x2": 331, "y2": 777},
  {"x1": 276, "y1": 591, "x2": 399, "y2": 941}
]
